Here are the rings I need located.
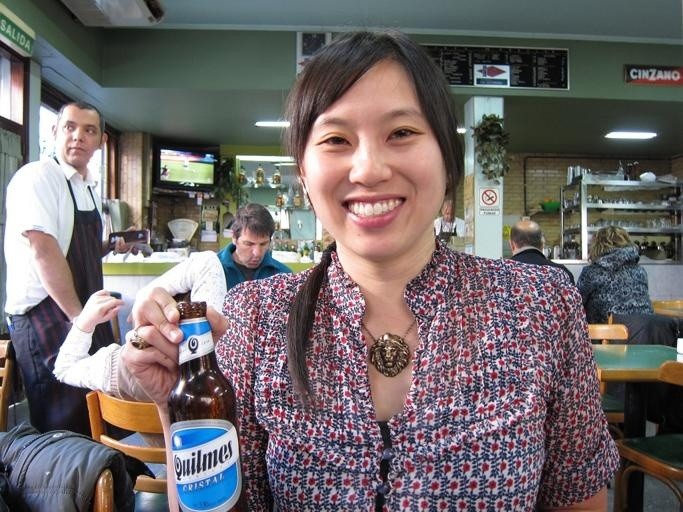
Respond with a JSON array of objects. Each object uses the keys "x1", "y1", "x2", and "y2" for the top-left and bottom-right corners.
[{"x1": 130, "y1": 325, "x2": 151, "y2": 349}]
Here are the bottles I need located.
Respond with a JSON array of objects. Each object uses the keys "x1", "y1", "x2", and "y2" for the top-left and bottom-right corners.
[
  {"x1": 293, "y1": 191, "x2": 302, "y2": 208},
  {"x1": 256, "y1": 163, "x2": 263, "y2": 184},
  {"x1": 238, "y1": 165, "x2": 244, "y2": 184},
  {"x1": 167, "y1": 301, "x2": 248, "y2": 512},
  {"x1": 275, "y1": 188, "x2": 282, "y2": 207},
  {"x1": 272, "y1": 166, "x2": 280, "y2": 184},
  {"x1": 564, "y1": 236, "x2": 580, "y2": 259},
  {"x1": 273, "y1": 236, "x2": 321, "y2": 260}
]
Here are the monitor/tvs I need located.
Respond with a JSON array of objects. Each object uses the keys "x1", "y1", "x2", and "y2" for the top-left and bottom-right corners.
[{"x1": 152, "y1": 137, "x2": 221, "y2": 192}]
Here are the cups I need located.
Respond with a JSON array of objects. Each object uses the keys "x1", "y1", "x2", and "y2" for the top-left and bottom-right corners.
[
  {"x1": 564, "y1": 193, "x2": 681, "y2": 234},
  {"x1": 566, "y1": 165, "x2": 590, "y2": 185},
  {"x1": 552, "y1": 245, "x2": 560, "y2": 260}
]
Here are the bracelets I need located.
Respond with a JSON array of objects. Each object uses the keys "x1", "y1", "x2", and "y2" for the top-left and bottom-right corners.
[{"x1": 72, "y1": 315, "x2": 95, "y2": 333}]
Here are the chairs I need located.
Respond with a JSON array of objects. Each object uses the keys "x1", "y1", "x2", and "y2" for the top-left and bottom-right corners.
[
  {"x1": 0, "y1": 296, "x2": 183, "y2": 510},
  {"x1": 577, "y1": 297, "x2": 683, "y2": 509}
]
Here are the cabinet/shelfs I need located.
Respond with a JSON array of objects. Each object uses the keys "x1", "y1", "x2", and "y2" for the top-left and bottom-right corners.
[{"x1": 562, "y1": 172, "x2": 679, "y2": 264}]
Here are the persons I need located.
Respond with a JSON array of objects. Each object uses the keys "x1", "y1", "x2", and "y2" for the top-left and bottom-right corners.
[
  {"x1": 3, "y1": 101, "x2": 137, "y2": 432},
  {"x1": 434, "y1": 199, "x2": 464, "y2": 243},
  {"x1": 53, "y1": 249, "x2": 228, "y2": 469},
  {"x1": 510, "y1": 220, "x2": 575, "y2": 285},
  {"x1": 217, "y1": 202, "x2": 293, "y2": 292},
  {"x1": 577, "y1": 226, "x2": 655, "y2": 324},
  {"x1": 122, "y1": 31, "x2": 621, "y2": 512}
]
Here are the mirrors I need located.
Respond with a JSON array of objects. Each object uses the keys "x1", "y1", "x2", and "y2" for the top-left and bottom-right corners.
[{"x1": 238, "y1": 155, "x2": 316, "y2": 251}]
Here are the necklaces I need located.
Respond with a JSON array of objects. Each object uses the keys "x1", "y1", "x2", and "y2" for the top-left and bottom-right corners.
[{"x1": 361, "y1": 319, "x2": 416, "y2": 377}]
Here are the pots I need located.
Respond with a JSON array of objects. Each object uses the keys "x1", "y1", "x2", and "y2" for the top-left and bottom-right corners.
[{"x1": 645, "y1": 246, "x2": 674, "y2": 259}]
